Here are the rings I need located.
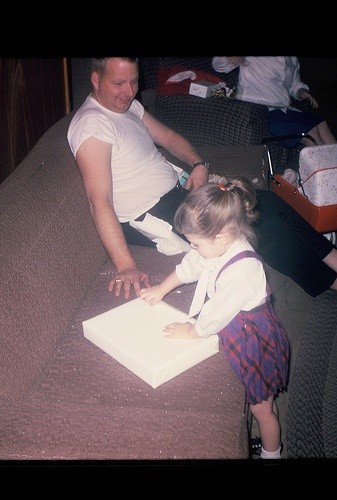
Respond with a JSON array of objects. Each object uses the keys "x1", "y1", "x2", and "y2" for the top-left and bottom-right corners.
[{"x1": 114, "y1": 277, "x2": 122, "y2": 283}]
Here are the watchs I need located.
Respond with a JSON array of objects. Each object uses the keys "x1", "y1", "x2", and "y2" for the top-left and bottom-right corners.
[{"x1": 192, "y1": 160, "x2": 211, "y2": 170}]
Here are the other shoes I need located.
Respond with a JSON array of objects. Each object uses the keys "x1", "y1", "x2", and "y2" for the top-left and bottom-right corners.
[{"x1": 250, "y1": 437, "x2": 283, "y2": 455}]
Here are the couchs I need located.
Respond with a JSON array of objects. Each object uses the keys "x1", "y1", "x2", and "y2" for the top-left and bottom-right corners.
[
  {"x1": 0, "y1": 106, "x2": 265, "y2": 460},
  {"x1": 140, "y1": 53, "x2": 320, "y2": 146}
]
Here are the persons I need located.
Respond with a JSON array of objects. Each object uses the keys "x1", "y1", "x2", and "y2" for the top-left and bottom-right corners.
[
  {"x1": 67, "y1": 56, "x2": 337, "y2": 300},
  {"x1": 211, "y1": 56, "x2": 337, "y2": 147},
  {"x1": 139, "y1": 176, "x2": 291, "y2": 459}
]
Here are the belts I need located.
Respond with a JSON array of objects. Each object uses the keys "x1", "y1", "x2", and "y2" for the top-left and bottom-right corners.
[{"x1": 160, "y1": 180, "x2": 181, "y2": 201}]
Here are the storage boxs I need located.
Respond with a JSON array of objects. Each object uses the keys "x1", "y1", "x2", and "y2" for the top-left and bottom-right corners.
[
  {"x1": 82, "y1": 296, "x2": 220, "y2": 388},
  {"x1": 189, "y1": 80, "x2": 222, "y2": 99}
]
